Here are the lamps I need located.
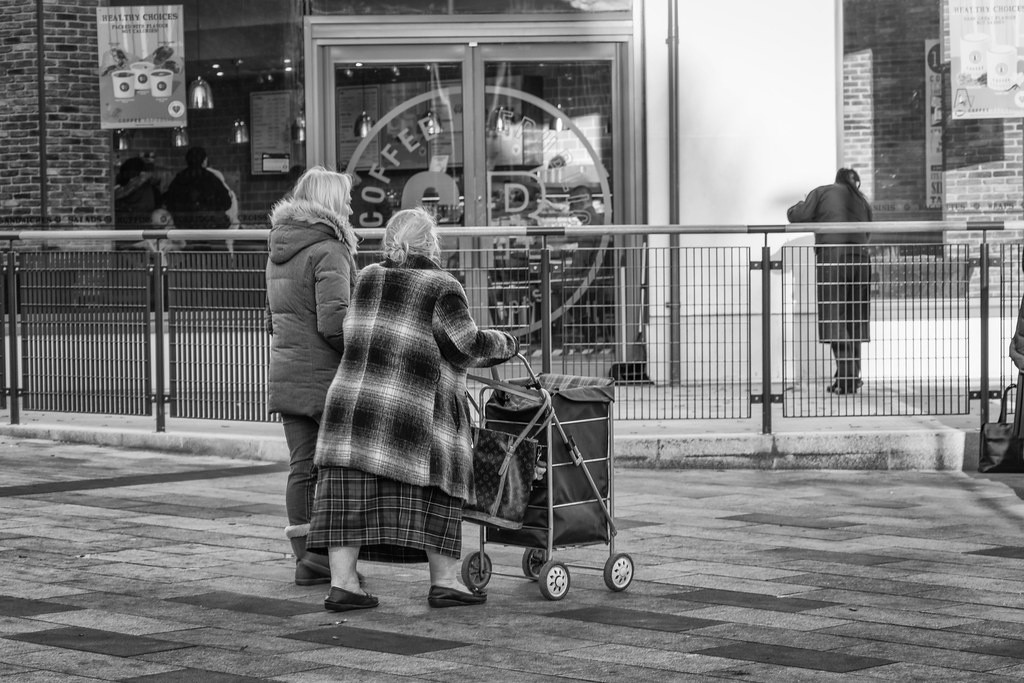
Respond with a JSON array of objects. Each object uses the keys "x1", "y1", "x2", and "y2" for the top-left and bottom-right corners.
[
  {"x1": 492, "y1": 105, "x2": 517, "y2": 140},
  {"x1": 352, "y1": 71, "x2": 376, "y2": 137},
  {"x1": 415, "y1": 68, "x2": 442, "y2": 134},
  {"x1": 112, "y1": 127, "x2": 130, "y2": 152},
  {"x1": 290, "y1": 107, "x2": 306, "y2": 145},
  {"x1": 184, "y1": 0, "x2": 215, "y2": 110},
  {"x1": 226, "y1": 60, "x2": 248, "y2": 145},
  {"x1": 170, "y1": 125, "x2": 189, "y2": 148}
]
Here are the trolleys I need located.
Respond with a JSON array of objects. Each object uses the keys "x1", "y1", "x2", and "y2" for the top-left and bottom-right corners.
[{"x1": 460, "y1": 351, "x2": 635, "y2": 601}]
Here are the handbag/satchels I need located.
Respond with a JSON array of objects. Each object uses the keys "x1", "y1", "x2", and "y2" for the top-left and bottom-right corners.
[
  {"x1": 978, "y1": 385, "x2": 1024, "y2": 472},
  {"x1": 462, "y1": 426, "x2": 538, "y2": 533}
]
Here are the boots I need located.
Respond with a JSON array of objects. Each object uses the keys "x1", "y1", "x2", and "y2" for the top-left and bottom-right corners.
[
  {"x1": 285, "y1": 523, "x2": 331, "y2": 585},
  {"x1": 301, "y1": 551, "x2": 365, "y2": 585}
]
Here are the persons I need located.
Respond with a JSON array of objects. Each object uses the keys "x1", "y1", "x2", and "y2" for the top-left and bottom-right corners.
[
  {"x1": 1008, "y1": 246, "x2": 1024, "y2": 376},
  {"x1": 114, "y1": 156, "x2": 165, "y2": 253},
  {"x1": 164, "y1": 145, "x2": 240, "y2": 251},
  {"x1": 311, "y1": 207, "x2": 521, "y2": 610},
  {"x1": 263, "y1": 165, "x2": 369, "y2": 587},
  {"x1": 786, "y1": 168, "x2": 873, "y2": 393}
]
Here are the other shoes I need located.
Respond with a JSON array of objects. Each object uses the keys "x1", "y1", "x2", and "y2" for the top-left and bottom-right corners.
[
  {"x1": 827, "y1": 379, "x2": 857, "y2": 394},
  {"x1": 324, "y1": 586, "x2": 379, "y2": 611},
  {"x1": 428, "y1": 585, "x2": 487, "y2": 607}
]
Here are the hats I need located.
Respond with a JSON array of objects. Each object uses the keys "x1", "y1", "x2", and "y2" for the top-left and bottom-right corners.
[
  {"x1": 186, "y1": 146, "x2": 206, "y2": 164},
  {"x1": 151, "y1": 208, "x2": 171, "y2": 224}
]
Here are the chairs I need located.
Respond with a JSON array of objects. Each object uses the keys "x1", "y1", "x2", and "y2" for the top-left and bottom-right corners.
[{"x1": 448, "y1": 236, "x2": 614, "y2": 348}]
